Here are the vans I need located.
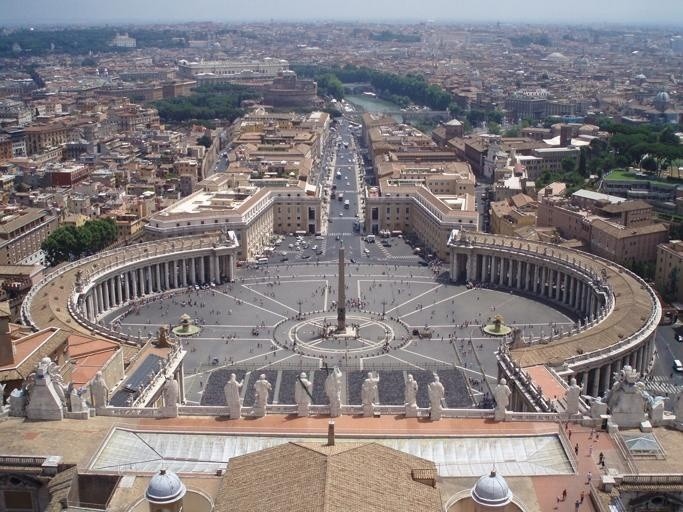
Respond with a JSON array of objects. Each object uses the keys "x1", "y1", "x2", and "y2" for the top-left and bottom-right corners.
[{"x1": 672, "y1": 359, "x2": 682, "y2": 372}]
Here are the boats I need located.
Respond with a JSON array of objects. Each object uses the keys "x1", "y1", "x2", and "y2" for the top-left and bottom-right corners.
[{"x1": 360, "y1": 91, "x2": 377, "y2": 101}]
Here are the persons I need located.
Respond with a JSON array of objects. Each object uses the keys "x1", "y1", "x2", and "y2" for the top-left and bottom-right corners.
[
  {"x1": 89, "y1": 261, "x2": 604, "y2": 512},
  {"x1": 644, "y1": 350, "x2": 680, "y2": 401}
]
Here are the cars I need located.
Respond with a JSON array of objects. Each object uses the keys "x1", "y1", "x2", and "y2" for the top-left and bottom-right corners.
[
  {"x1": 674, "y1": 333, "x2": 682, "y2": 341},
  {"x1": 254, "y1": 228, "x2": 326, "y2": 265},
  {"x1": 363, "y1": 230, "x2": 433, "y2": 267},
  {"x1": 331, "y1": 135, "x2": 356, "y2": 217},
  {"x1": 334, "y1": 235, "x2": 340, "y2": 240},
  {"x1": 350, "y1": 258, "x2": 355, "y2": 264},
  {"x1": 215, "y1": 151, "x2": 227, "y2": 164}
]
